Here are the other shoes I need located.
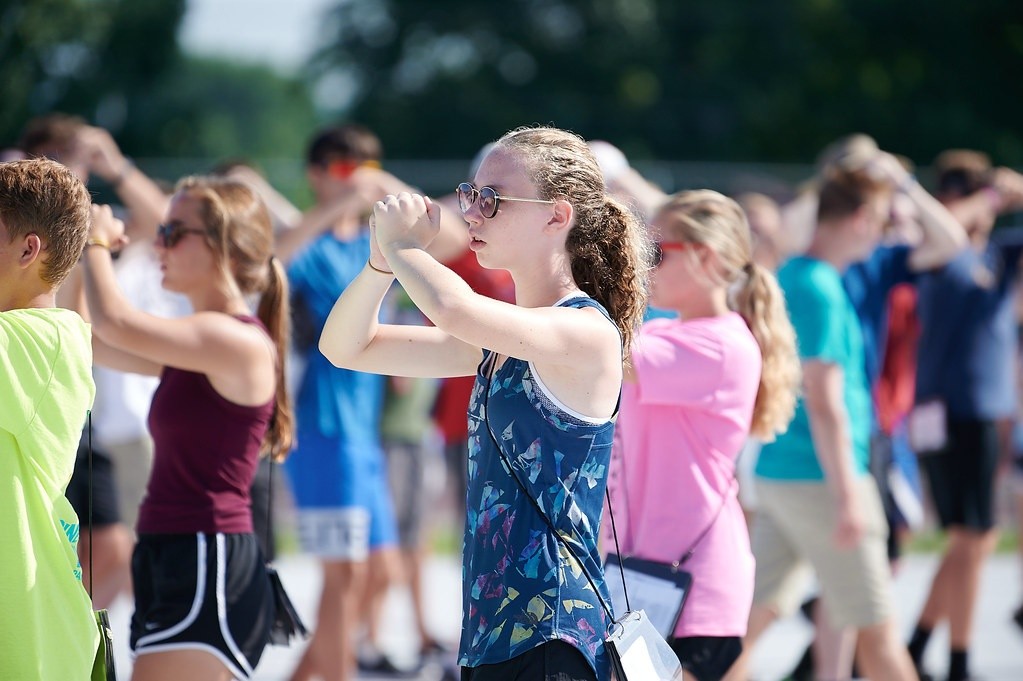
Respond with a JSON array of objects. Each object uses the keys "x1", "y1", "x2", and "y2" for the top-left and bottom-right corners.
[
  {"x1": 357, "y1": 655, "x2": 404, "y2": 677},
  {"x1": 420, "y1": 645, "x2": 451, "y2": 665}
]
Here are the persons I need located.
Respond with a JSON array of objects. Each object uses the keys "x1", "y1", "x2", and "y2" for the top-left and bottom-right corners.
[
  {"x1": 732, "y1": 136, "x2": 1023, "y2": 679},
  {"x1": 70, "y1": 175, "x2": 298, "y2": 681},
  {"x1": 0, "y1": 118, "x2": 473, "y2": 680},
  {"x1": 749, "y1": 155, "x2": 921, "y2": 681},
  {"x1": 0, "y1": 156, "x2": 102, "y2": 681},
  {"x1": 318, "y1": 127, "x2": 645, "y2": 681},
  {"x1": 600, "y1": 189, "x2": 805, "y2": 681}
]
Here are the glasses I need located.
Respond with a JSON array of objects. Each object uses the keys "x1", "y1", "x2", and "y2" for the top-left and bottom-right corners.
[
  {"x1": 456, "y1": 183, "x2": 557, "y2": 219},
  {"x1": 158, "y1": 219, "x2": 211, "y2": 249},
  {"x1": 644, "y1": 242, "x2": 700, "y2": 266}
]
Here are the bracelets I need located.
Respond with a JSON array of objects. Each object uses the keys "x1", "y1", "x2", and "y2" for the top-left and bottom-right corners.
[
  {"x1": 85, "y1": 239, "x2": 111, "y2": 249},
  {"x1": 368, "y1": 258, "x2": 394, "y2": 274}
]
